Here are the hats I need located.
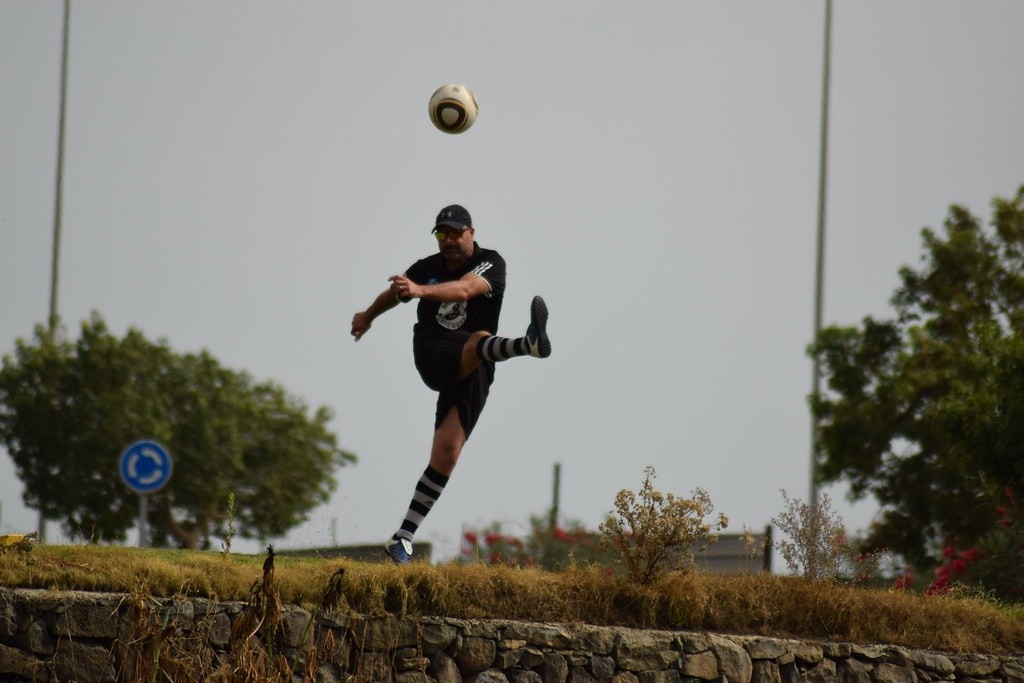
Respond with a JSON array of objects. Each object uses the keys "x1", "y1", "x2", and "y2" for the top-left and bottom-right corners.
[{"x1": 431, "y1": 205, "x2": 472, "y2": 234}]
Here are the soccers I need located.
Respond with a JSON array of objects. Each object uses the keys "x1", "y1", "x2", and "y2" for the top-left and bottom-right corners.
[{"x1": 427, "y1": 83, "x2": 480, "y2": 135}]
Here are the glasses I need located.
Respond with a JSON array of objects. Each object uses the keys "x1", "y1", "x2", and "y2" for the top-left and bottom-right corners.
[{"x1": 434, "y1": 228, "x2": 471, "y2": 242}]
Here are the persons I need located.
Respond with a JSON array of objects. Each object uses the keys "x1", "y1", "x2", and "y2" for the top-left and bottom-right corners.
[{"x1": 350, "y1": 205, "x2": 550, "y2": 565}]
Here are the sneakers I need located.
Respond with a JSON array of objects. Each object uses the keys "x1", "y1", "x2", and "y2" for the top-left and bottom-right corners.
[
  {"x1": 526, "y1": 295, "x2": 551, "y2": 358},
  {"x1": 384, "y1": 534, "x2": 413, "y2": 567}
]
